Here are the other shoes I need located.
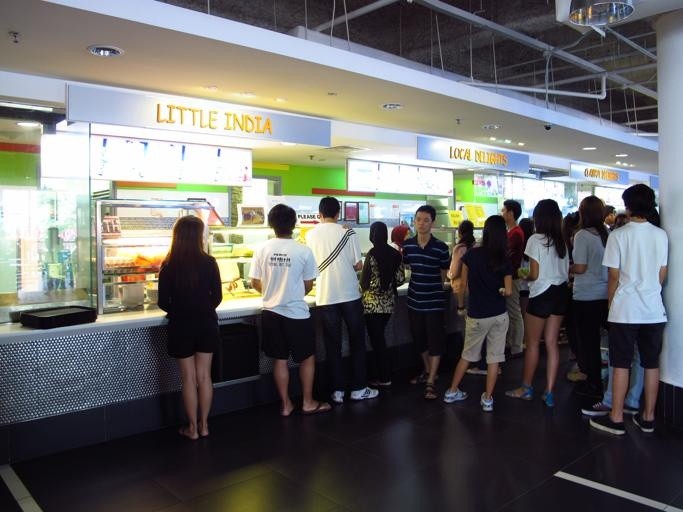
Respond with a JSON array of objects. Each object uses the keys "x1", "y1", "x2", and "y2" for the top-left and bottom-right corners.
[
  {"x1": 567, "y1": 370, "x2": 588, "y2": 382},
  {"x1": 367, "y1": 380, "x2": 392, "y2": 386},
  {"x1": 425, "y1": 381, "x2": 438, "y2": 401},
  {"x1": 409, "y1": 372, "x2": 440, "y2": 386},
  {"x1": 466, "y1": 366, "x2": 502, "y2": 375}
]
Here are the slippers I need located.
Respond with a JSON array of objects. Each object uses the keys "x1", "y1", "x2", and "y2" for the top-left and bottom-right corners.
[
  {"x1": 283, "y1": 402, "x2": 301, "y2": 418},
  {"x1": 177, "y1": 424, "x2": 209, "y2": 441},
  {"x1": 304, "y1": 402, "x2": 330, "y2": 415}
]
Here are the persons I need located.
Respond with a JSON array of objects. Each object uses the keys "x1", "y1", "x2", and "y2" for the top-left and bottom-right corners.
[
  {"x1": 247, "y1": 203, "x2": 335, "y2": 421},
  {"x1": 400, "y1": 205, "x2": 451, "y2": 400},
  {"x1": 305, "y1": 195, "x2": 380, "y2": 404},
  {"x1": 358, "y1": 221, "x2": 405, "y2": 388},
  {"x1": 156, "y1": 214, "x2": 223, "y2": 441},
  {"x1": 503, "y1": 183, "x2": 669, "y2": 435},
  {"x1": 389, "y1": 225, "x2": 411, "y2": 286},
  {"x1": 445, "y1": 219, "x2": 503, "y2": 377},
  {"x1": 443, "y1": 215, "x2": 514, "y2": 413}
]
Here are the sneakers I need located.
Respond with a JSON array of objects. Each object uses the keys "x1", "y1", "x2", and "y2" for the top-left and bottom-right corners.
[
  {"x1": 581, "y1": 401, "x2": 611, "y2": 416},
  {"x1": 622, "y1": 405, "x2": 638, "y2": 415},
  {"x1": 332, "y1": 391, "x2": 344, "y2": 403},
  {"x1": 350, "y1": 387, "x2": 379, "y2": 400},
  {"x1": 590, "y1": 415, "x2": 626, "y2": 435},
  {"x1": 480, "y1": 391, "x2": 494, "y2": 412},
  {"x1": 444, "y1": 388, "x2": 469, "y2": 402},
  {"x1": 632, "y1": 413, "x2": 654, "y2": 433}
]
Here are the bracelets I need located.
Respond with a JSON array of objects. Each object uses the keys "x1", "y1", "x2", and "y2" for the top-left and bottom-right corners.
[{"x1": 456, "y1": 305, "x2": 465, "y2": 310}]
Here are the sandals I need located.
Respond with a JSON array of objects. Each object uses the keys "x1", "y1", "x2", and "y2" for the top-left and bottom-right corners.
[
  {"x1": 538, "y1": 390, "x2": 555, "y2": 407},
  {"x1": 504, "y1": 382, "x2": 532, "y2": 400}
]
